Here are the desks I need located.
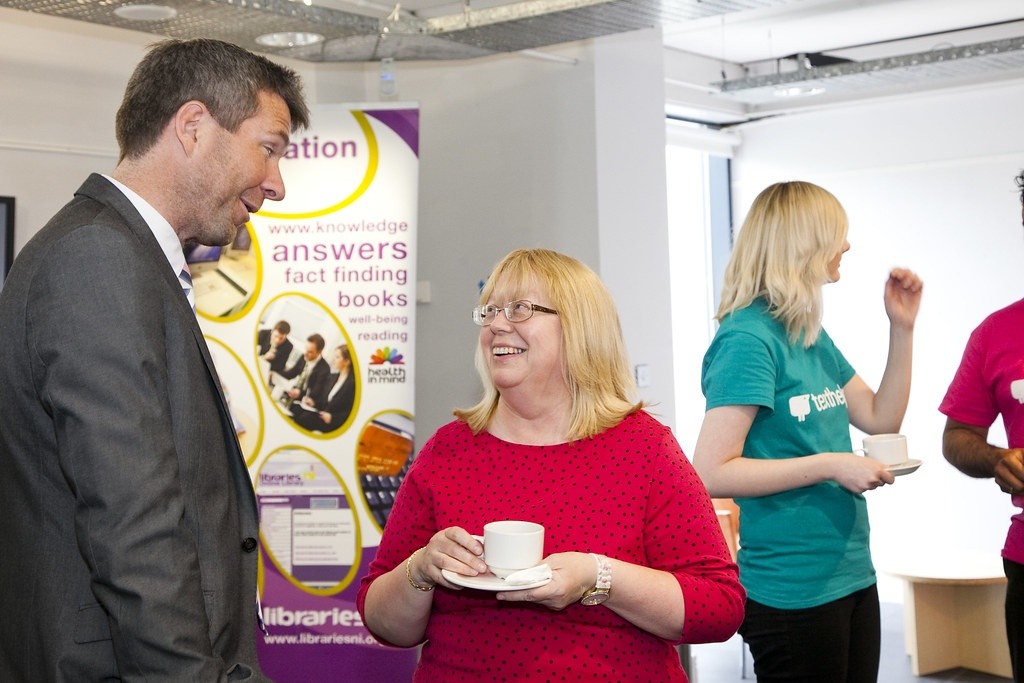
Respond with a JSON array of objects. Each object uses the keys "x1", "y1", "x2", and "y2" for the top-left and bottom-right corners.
[{"x1": 895, "y1": 572, "x2": 1013, "y2": 679}]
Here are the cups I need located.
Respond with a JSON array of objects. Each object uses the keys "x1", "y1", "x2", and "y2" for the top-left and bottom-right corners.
[
  {"x1": 854, "y1": 434, "x2": 908, "y2": 466},
  {"x1": 471, "y1": 521, "x2": 544, "y2": 579}
]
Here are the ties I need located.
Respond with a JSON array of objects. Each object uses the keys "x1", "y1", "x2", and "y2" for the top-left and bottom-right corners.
[{"x1": 180, "y1": 263, "x2": 196, "y2": 319}]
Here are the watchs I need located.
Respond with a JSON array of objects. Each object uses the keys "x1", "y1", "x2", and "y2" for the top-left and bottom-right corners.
[{"x1": 579, "y1": 553, "x2": 613, "y2": 607}]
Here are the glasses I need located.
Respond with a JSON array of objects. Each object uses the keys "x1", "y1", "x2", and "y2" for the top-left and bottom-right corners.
[{"x1": 472, "y1": 300, "x2": 559, "y2": 327}]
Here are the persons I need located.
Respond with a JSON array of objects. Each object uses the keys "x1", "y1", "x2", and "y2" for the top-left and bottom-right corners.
[
  {"x1": 692, "y1": 177, "x2": 926, "y2": 683},
  {"x1": 352, "y1": 247, "x2": 748, "y2": 682},
  {"x1": 3, "y1": 35, "x2": 315, "y2": 681},
  {"x1": 936, "y1": 165, "x2": 1024, "y2": 682},
  {"x1": 257, "y1": 319, "x2": 356, "y2": 432}
]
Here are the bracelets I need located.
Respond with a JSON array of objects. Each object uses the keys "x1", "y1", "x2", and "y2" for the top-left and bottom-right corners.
[{"x1": 404, "y1": 546, "x2": 436, "y2": 592}]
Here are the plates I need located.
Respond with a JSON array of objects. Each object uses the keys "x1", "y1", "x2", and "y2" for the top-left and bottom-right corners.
[
  {"x1": 887, "y1": 460, "x2": 923, "y2": 476},
  {"x1": 441, "y1": 568, "x2": 551, "y2": 592}
]
